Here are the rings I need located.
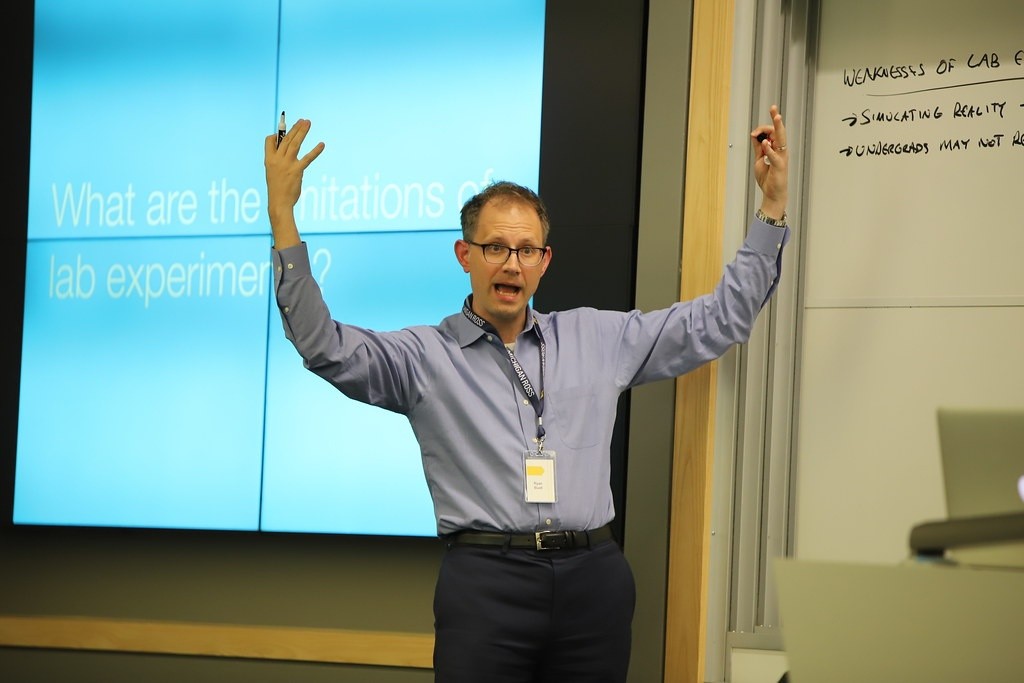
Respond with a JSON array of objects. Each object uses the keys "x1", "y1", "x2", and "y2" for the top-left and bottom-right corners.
[{"x1": 773, "y1": 145, "x2": 787, "y2": 151}]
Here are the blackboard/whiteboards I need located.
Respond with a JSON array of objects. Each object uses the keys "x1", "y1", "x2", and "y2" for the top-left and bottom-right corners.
[{"x1": 758, "y1": 0, "x2": 1024, "y2": 628}]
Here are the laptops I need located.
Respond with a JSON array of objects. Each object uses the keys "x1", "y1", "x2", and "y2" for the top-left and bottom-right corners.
[{"x1": 937, "y1": 405, "x2": 1024, "y2": 569}]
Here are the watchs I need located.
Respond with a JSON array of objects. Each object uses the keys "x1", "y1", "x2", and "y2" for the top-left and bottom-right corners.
[{"x1": 755, "y1": 209, "x2": 788, "y2": 227}]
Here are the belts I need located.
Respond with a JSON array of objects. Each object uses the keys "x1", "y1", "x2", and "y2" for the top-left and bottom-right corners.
[{"x1": 447, "y1": 523, "x2": 613, "y2": 552}]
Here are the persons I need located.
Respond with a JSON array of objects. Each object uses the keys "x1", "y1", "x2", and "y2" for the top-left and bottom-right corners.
[{"x1": 264, "y1": 104, "x2": 793, "y2": 683}]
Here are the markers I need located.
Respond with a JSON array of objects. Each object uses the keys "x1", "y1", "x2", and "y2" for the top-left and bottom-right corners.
[{"x1": 277, "y1": 111, "x2": 287, "y2": 149}]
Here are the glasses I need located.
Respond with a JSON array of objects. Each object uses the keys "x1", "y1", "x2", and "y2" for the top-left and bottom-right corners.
[{"x1": 464, "y1": 237, "x2": 547, "y2": 267}]
